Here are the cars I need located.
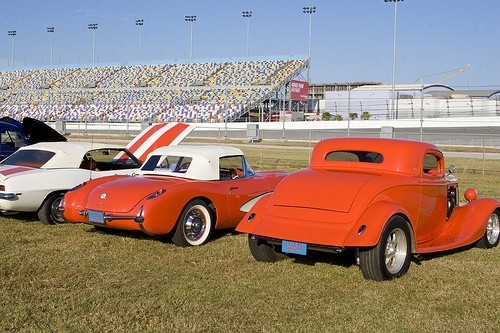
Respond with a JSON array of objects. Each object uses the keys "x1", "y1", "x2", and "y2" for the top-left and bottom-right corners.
[
  {"x1": 0, "y1": 117, "x2": 198, "y2": 226},
  {"x1": 58, "y1": 144, "x2": 288, "y2": 249},
  {"x1": 235, "y1": 137, "x2": 500, "y2": 281},
  {"x1": 1, "y1": 117, "x2": 68, "y2": 164}
]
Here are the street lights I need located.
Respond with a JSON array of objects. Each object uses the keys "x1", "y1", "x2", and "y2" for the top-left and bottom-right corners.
[
  {"x1": 135, "y1": 20, "x2": 144, "y2": 56},
  {"x1": 7, "y1": 30, "x2": 16, "y2": 66},
  {"x1": 384, "y1": 0, "x2": 405, "y2": 120},
  {"x1": 241, "y1": 10, "x2": 253, "y2": 57},
  {"x1": 184, "y1": 15, "x2": 197, "y2": 63},
  {"x1": 46, "y1": 26, "x2": 56, "y2": 67},
  {"x1": 302, "y1": 5, "x2": 316, "y2": 83},
  {"x1": 88, "y1": 23, "x2": 98, "y2": 68}
]
